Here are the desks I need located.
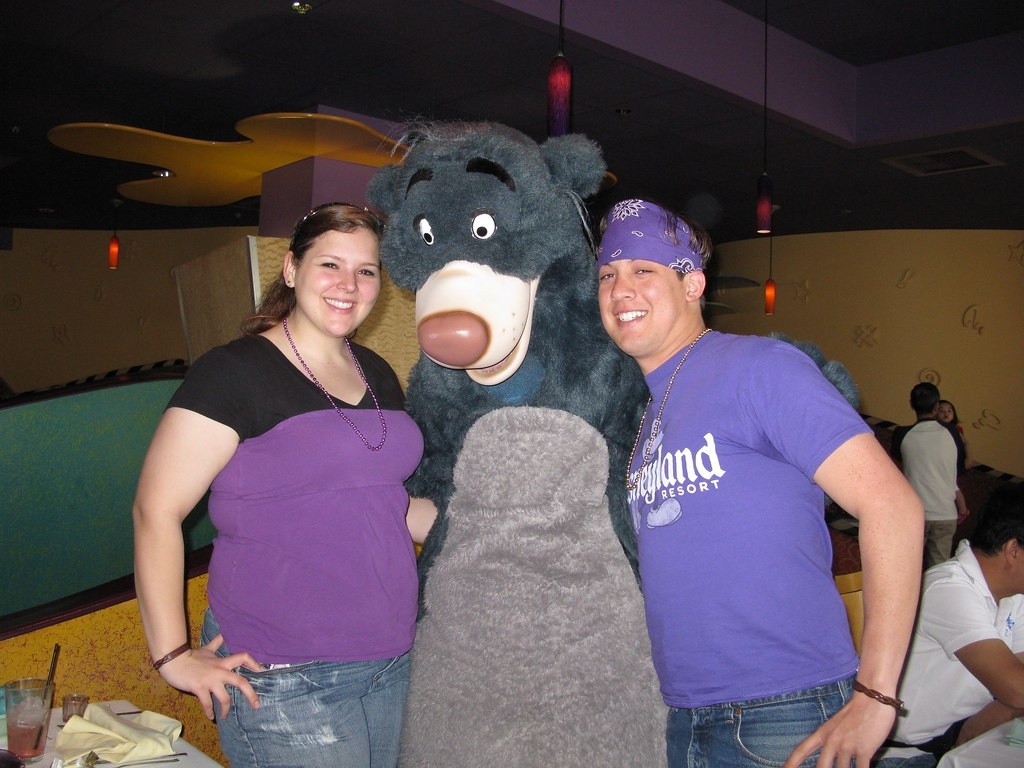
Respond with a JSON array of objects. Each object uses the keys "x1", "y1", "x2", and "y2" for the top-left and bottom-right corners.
[
  {"x1": 0, "y1": 700, "x2": 223, "y2": 768},
  {"x1": 936, "y1": 716, "x2": 1024, "y2": 768}
]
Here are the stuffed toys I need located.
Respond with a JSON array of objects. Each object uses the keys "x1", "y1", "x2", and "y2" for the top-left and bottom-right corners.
[{"x1": 365, "y1": 105, "x2": 608, "y2": 768}]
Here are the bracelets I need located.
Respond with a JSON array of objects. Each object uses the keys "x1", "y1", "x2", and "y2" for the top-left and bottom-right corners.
[
  {"x1": 852, "y1": 680, "x2": 904, "y2": 710},
  {"x1": 153, "y1": 641, "x2": 190, "y2": 670}
]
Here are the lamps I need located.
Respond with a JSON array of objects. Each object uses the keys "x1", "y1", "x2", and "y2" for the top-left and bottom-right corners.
[
  {"x1": 545, "y1": 0, "x2": 571, "y2": 137},
  {"x1": 107, "y1": 230, "x2": 120, "y2": 270},
  {"x1": 762, "y1": 222, "x2": 777, "y2": 316},
  {"x1": 756, "y1": 0, "x2": 773, "y2": 234}
]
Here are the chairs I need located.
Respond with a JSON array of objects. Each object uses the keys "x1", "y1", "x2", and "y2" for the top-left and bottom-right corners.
[{"x1": 834, "y1": 570, "x2": 865, "y2": 656}]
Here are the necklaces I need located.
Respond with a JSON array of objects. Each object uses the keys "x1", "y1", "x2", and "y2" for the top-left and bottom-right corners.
[
  {"x1": 283, "y1": 318, "x2": 387, "y2": 451},
  {"x1": 626, "y1": 328, "x2": 712, "y2": 490}
]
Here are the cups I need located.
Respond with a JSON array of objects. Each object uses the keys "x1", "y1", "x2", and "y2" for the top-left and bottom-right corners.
[
  {"x1": 0, "y1": 685, "x2": 26, "y2": 768},
  {"x1": 5, "y1": 679, "x2": 55, "y2": 763},
  {"x1": 62, "y1": 693, "x2": 88, "y2": 722}
]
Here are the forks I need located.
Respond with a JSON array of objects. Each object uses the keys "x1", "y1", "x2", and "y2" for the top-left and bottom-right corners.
[{"x1": 85, "y1": 750, "x2": 179, "y2": 768}]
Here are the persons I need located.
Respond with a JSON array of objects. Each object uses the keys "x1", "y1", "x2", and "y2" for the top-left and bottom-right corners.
[
  {"x1": 888, "y1": 482, "x2": 1024, "y2": 754},
  {"x1": 594, "y1": 192, "x2": 925, "y2": 767},
  {"x1": 132, "y1": 201, "x2": 438, "y2": 768},
  {"x1": 891, "y1": 382, "x2": 972, "y2": 570}
]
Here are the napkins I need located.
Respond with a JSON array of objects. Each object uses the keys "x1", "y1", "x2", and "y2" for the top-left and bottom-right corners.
[
  {"x1": 55, "y1": 701, "x2": 177, "y2": 764},
  {"x1": 1003, "y1": 717, "x2": 1024, "y2": 748},
  {"x1": 130, "y1": 709, "x2": 182, "y2": 746}
]
